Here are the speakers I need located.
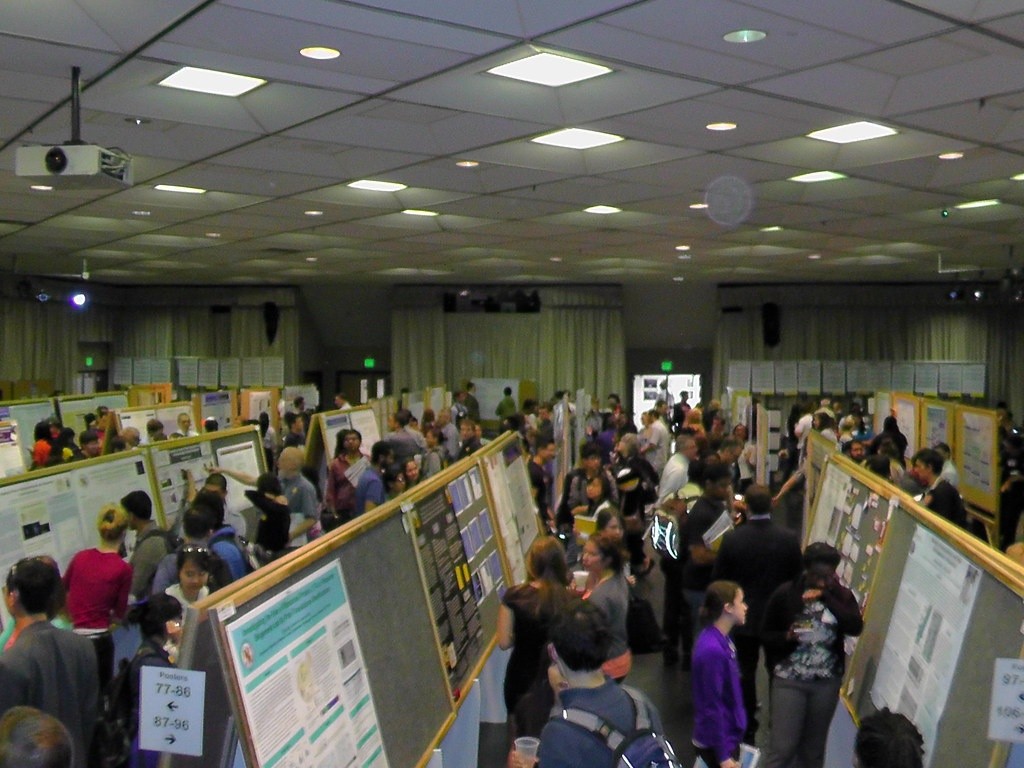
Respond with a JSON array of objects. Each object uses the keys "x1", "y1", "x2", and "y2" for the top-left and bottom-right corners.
[
  {"x1": 762, "y1": 301, "x2": 781, "y2": 347},
  {"x1": 265, "y1": 302, "x2": 280, "y2": 345}
]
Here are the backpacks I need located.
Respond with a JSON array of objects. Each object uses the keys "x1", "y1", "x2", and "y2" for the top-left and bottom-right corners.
[
  {"x1": 90, "y1": 647, "x2": 169, "y2": 768},
  {"x1": 649, "y1": 490, "x2": 700, "y2": 559},
  {"x1": 208, "y1": 533, "x2": 276, "y2": 570},
  {"x1": 137, "y1": 528, "x2": 185, "y2": 548},
  {"x1": 550, "y1": 684, "x2": 683, "y2": 768}
]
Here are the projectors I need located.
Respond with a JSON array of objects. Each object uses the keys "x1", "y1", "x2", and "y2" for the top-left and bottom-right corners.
[{"x1": 15, "y1": 144, "x2": 133, "y2": 191}]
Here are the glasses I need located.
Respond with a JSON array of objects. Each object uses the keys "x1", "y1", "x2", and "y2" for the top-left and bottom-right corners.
[{"x1": 181, "y1": 546, "x2": 208, "y2": 553}]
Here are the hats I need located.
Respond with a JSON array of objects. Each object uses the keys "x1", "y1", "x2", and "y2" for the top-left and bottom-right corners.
[
  {"x1": 802, "y1": 542, "x2": 840, "y2": 575},
  {"x1": 118, "y1": 491, "x2": 152, "y2": 519}
]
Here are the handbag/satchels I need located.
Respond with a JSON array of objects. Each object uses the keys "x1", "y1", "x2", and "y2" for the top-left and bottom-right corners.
[
  {"x1": 624, "y1": 576, "x2": 663, "y2": 657},
  {"x1": 623, "y1": 514, "x2": 645, "y2": 532}
]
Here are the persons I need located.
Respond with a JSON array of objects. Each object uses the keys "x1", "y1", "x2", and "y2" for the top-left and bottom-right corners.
[
  {"x1": 972, "y1": 406, "x2": 1024, "y2": 553},
  {"x1": 1, "y1": 382, "x2": 482, "y2": 768},
  {"x1": 771, "y1": 397, "x2": 967, "y2": 529},
  {"x1": 764, "y1": 542, "x2": 864, "y2": 768},
  {"x1": 509, "y1": 602, "x2": 662, "y2": 768},
  {"x1": 495, "y1": 387, "x2": 803, "y2": 747},
  {"x1": 852, "y1": 707, "x2": 924, "y2": 768},
  {"x1": 690, "y1": 581, "x2": 748, "y2": 768},
  {"x1": 502, "y1": 538, "x2": 580, "y2": 746}
]
